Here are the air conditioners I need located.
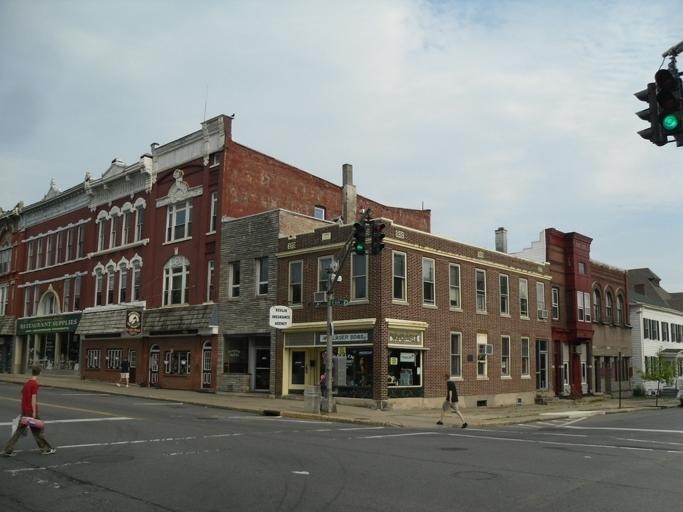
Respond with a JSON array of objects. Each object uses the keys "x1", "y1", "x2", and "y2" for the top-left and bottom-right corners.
[
  {"x1": 537, "y1": 310, "x2": 549, "y2": 319},
  {"x1": 313, "y1": 290, "x2": 327, "y2": 304},
  {"x1": 481, "y1": 344, "x2": 494, "y2": 356}
]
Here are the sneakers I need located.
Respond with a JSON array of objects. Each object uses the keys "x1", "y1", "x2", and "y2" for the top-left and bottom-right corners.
[
  {"x1": 437, "y1": 421, "x2": 443, "y2": 425},
  {"x1": 41, "y1": 448, "x2": 55, "y2": 455},
  {"x1": 4, "y1": 452, "x2": 16, "y2": 456},
  {"x1": 461, "y1": 423, "x2": 468, "y2": 428}
]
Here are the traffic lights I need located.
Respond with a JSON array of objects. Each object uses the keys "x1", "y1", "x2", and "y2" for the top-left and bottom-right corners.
[
  {"x1": 633, "y1": 83, "x2": 656, "y2": 142},
  {"x1": 352, "y1": 222, "x2": 366, "y2": 256},
  {"x1": 654, "y1": 69, "x2": 683, "y2": 146},
  {"x1": 371, "y1": 223, "x2": 385, "y2": 255}
]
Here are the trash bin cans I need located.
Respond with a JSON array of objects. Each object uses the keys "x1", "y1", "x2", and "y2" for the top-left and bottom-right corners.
[
  {"x1": 304, "y1": 385, "x2": 322, "y2": 414},
  {"x1": 74, "y1": 363, "x2": 80, "y2": 376}
]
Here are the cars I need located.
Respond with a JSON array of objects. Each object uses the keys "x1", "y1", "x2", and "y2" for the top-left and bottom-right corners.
[{"x1": 676, "y1": 376, "x2": 683, "y2": 407}]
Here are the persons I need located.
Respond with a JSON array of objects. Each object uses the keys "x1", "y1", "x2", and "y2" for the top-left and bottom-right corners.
[
  {"x1": 116, "y1": 356, "x2": 130, "y2": 387},
  {"x1": 437, "y1": 373, "x2": 467, "y2": 428},
  {"x1": 0, "y1": 366, "x2": 56, "y2": 457}
]
